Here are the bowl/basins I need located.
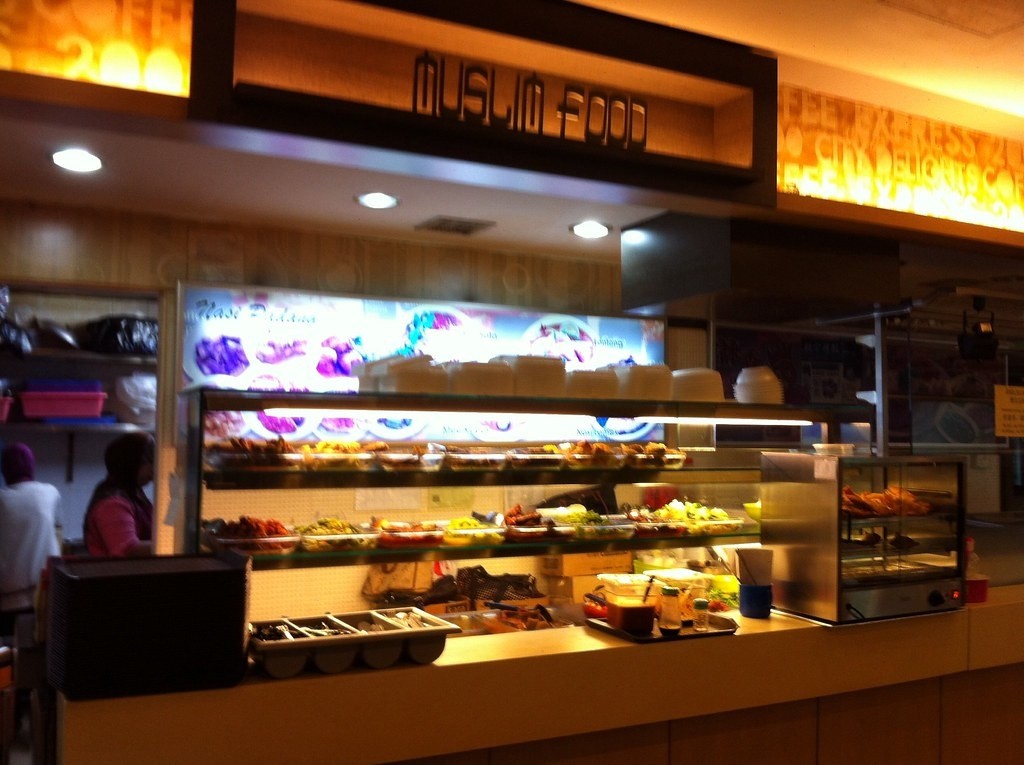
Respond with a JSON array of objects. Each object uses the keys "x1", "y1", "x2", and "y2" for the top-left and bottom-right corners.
[
  {"x1": 964, "y1": 575, "x2": 990, "y2": 603},
  {"x1": 732, "y1": 366, "x2": 785, "y2": 404},
  {"x1": 744, "y1": 503, "x2": 761, "y2": 523}
]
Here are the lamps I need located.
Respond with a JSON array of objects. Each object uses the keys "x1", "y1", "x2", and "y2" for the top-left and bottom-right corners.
[{"x1": 956, "y1": 296, "x2": 1000, "y2": 362}]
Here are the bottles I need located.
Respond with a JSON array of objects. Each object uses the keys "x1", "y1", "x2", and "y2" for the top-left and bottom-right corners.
[
  {"x1": 966, "y1": 533, "x2": 981, "y2": 578},
  {"x1": 658, "y1": 587, "x2": 682, "y2": 638},
  {"x1": 693, "y1": 598, "x2": 708, "y2": 632}
]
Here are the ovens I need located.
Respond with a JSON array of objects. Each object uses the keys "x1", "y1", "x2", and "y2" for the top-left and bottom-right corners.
[{"x1": 761, "y1": 449, "x2": 966, "y2": 625}]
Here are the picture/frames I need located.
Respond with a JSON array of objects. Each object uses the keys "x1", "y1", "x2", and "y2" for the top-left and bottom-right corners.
[{"x1": 711, "y1": 318, "x2": 1010, "y2": 448}]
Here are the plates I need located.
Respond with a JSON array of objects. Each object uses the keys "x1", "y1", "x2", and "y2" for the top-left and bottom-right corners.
[{"x1": 812, "y1": 443, "x2": 857, "y2": 451}]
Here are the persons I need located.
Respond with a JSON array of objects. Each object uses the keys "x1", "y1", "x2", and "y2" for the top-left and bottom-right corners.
[
  {"x1": 0, "y1": 442, "x2": 64, "y2": 598},
  {"x1": 82, "y1": 429, "x2": 157, "y2": 557}
]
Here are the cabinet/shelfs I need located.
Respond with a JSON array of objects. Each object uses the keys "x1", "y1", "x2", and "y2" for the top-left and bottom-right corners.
[
  {"x1": 0, "y1": 348, "x2": 165, "y2": 435},
  {"x1": 171, "y1": 386, "x2": 875, "y2": 639}
]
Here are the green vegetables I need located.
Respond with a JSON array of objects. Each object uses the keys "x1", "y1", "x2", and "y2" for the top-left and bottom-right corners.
[
  {"x1": 553, "y1": 510, "x2": 627, "y2": 537},
  {"x1": 656, "y1": 499, "x2": 730, "y2": 522}
]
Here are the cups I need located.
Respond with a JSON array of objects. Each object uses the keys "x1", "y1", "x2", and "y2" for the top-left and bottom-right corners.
[{"x1": 739, "y1": 584, "x2": 773, "y2": 619}]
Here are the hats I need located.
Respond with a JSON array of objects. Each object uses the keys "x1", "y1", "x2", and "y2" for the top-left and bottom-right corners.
[
  {"x1": 0, "y1": 442, "x2": 35, "y2": 484},
  {"x1": 104, "y1": 432, "x2": 155, "y2": 479}
]
